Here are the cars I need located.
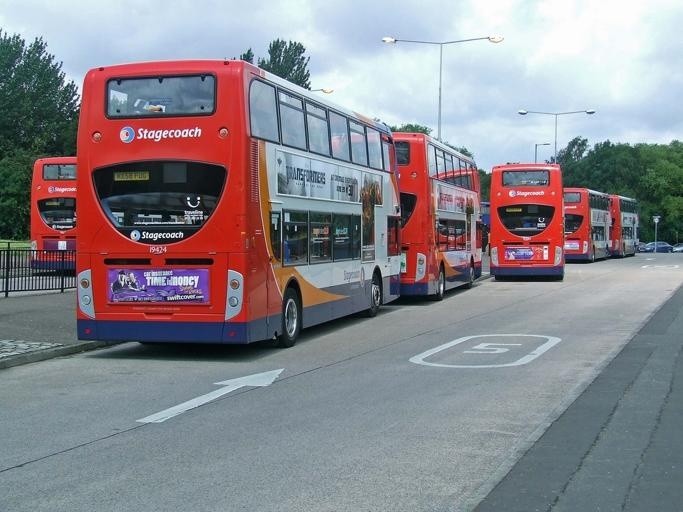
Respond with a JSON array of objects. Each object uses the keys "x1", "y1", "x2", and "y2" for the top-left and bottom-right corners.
[{"x1": 635, "y1": 241, "x2": 683, "y2": 253}]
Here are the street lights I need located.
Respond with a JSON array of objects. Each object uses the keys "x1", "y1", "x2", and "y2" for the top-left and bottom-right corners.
[
  {"x1": 653, "y1": 216, "x2": 661, "y2": 253},
  {"x1": 381, "y1": 35, "x2": 505, "y2": 142}
]
